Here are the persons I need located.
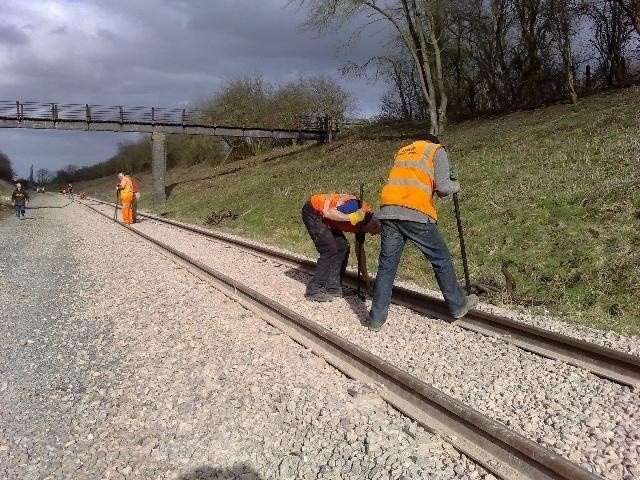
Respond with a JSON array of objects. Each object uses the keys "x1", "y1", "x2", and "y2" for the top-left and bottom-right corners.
[
  {"x1": 362, "y1": 134, "x2": 478, "y2": 332},
  {"x1": 115, "y1": 172, "x2": 134, "y2": 225},
  {"x1": 10, "y1": 182, "x2": 30, "y2": 218},
  {"x1": 124, "y1": 173, "x2": 140, "y2": 222},
  {"x1": 65, "y1": 182, "x2": 75, "y2": 198},
  {"x1": 300, "y1": 191, "x2": 382, "y2": 303}
]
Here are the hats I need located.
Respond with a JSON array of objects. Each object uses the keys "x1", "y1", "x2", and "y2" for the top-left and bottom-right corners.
[{"x1": 413, "y1": 133, "x2": 440, "y2": 144}]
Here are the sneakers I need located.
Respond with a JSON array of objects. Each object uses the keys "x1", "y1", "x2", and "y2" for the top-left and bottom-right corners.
[
  {"x1": 324, "y1": 286, "x2": 358, "y2": 298},
  {"x1": 306, "y1": 286, "x2": 333, "y2": 302},
  {"x1": 367, "y1": 315, "x2": 386, "y2": 332},
  {"x1": 449, "y1": 293, "x2": 479, "y2": 320}
]
